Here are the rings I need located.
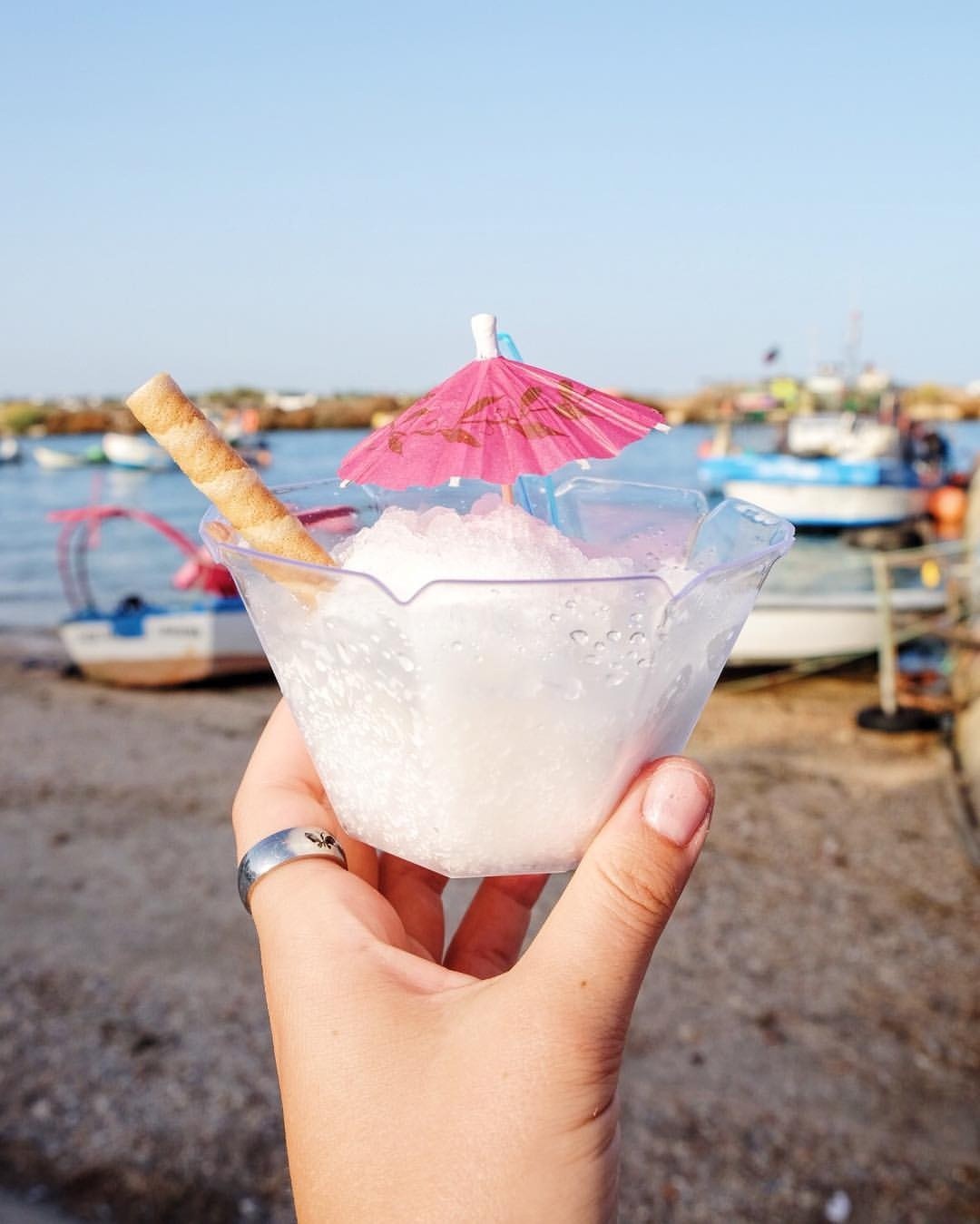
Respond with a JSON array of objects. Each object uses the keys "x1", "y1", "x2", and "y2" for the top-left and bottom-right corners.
[{"x1": 236, "y1": 825, "x2": 348, "y2": 915}]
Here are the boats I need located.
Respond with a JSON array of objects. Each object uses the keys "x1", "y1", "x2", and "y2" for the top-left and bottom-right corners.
[
  {"x1": 56, "y1": 597, "x2": 273, "y2": 685},
  {"x1": 695, "y1": 410, "x2": 962, "y2": 531},
  {"x1": 0, "y1": 429, "x2": 276, "y2": 471},
  {"x1": 723, "y1": 595, "x2": 949, "y2": 669}
]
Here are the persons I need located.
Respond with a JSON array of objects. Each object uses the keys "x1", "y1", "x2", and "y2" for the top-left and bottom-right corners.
[{"x1": 229, "y1": 692, "x2": 717, "y2": 1224}]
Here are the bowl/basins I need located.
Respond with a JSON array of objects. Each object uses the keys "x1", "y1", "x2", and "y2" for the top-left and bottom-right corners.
[{"x1": 197, "y1": 475, "x2": 797, "y2": 879}]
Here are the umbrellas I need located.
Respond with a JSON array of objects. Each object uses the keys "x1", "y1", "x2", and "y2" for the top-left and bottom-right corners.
[{"x1": 338, "y1": 314, "x2": 668, "y2": 508}]
